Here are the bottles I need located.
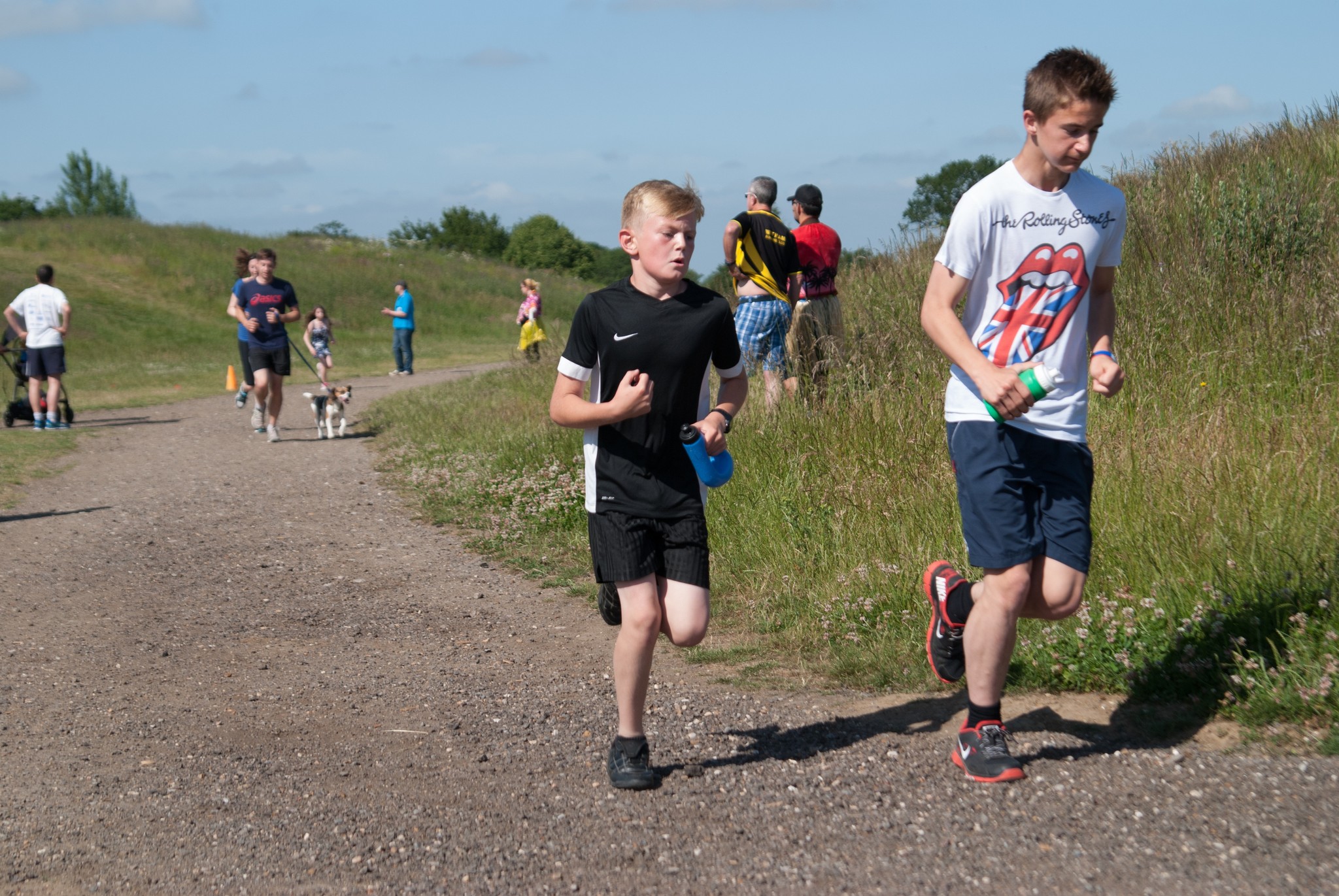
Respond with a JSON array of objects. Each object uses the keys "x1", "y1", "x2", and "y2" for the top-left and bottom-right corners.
[
  {"x1": 983, "y1": 364, "x2": 1064, "y2": 424},
  {"x1": 680, "y1": 424, "x2": 734, "y2": 488}
]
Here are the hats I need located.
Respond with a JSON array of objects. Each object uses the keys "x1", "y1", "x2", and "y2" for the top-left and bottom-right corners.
[
  {"x1": 393, "y1": 280, "x2": 407, "y2": 287},
  {"x1": 787, "y1": 184, "x2": 823, "y2": 205}
]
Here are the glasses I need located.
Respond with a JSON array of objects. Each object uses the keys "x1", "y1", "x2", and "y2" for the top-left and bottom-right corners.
[
  {"x1": 791, "y1": 199, "x2": 801, "y2": 205},
  {"x1": 744, "y1": 192, "x2": 757, "y2": 198}
]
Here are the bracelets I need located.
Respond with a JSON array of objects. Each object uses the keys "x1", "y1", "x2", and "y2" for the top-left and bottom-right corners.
[
  {"x1": 1089, "y1": 351, "x2": 1118, "y2": 364},
  {"x1": 274, "y1": 310, "x2": 281, "y2": 322}
]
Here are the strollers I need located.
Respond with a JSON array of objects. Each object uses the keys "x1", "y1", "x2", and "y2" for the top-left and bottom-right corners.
[{"x1": 0, "y1": 316, "x2": 74, "y2": 428}]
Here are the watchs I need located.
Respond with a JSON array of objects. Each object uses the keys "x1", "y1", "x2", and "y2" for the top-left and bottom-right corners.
[
  {"x1": 724, "y1": 258, "x2": 736, "y2": 267},
  {"x1": 708, "y1": 408, "x2": 734, "y2": 434}
]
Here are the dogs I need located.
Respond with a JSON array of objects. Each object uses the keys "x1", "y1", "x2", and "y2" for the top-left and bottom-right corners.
[{"x1": 299, "y1": 385, "x2": 353, "y2": 441}]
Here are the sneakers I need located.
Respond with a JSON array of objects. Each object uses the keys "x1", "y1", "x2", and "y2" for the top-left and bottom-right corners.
[
  {"x1": 266, "y1": 424, "x2": 280, "y2": 442},
  {"x1": 923, "y1": 559, "x2": 971, "y2": 684},
  {"x1": 597, "y1": 581, "x2": 623, "y2": 627},
  {"x1": 235, "y1": 383, "x2": 247, "y2": 408},
  {"x1": 32, "y1": 419, "x2": 46, "y2": 429},
  {"x1": 607, "y1": 735, "x2": 655, "y2": 789},
  {"x1": 951, "y1": 716, "x2": 1026, "y2": 782},
  {"x1": 251, "y1": 400, "x2": 266, "y2": 429},
  {"x1": 44, "y1": 418, "x2": 70, "y2": 429}
]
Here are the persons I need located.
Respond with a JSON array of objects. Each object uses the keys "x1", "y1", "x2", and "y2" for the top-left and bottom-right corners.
[
  {"x1": 722, "y1": 175, "x2": 805, "y2": 436},
  {"x1": 514, "y1": 278, "x2": 547, "y2": 366},
  {"x1": 785, "y1": 183, "x2": 842, "y2": 411},
  {"x1": 382, "y1": 279, "x2": 417, "y2": 376},
  {"x1": 921, "y1": 47, "x2": 1132, "y2": 783},
  {"x1": 547, "y1": 174, "x2": 748, "y2": 789},
  {"x1": 233, "y1": 247, "x2": 301, "y2": 443},
  {"x1": 226, "y1": 247, "x2": 268, "y2": 433},
  {"x1": 2, "y1": 262, "x2": 72, "y2": 431},
  {"x1": 301, "y1": 303, "x2": 337, "y2": 390}
]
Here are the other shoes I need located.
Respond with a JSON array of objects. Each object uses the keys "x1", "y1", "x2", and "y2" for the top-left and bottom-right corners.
[
  {"x1": 399, "y1": 370, "x2": 412, "y2": 374},
  {"x1": 321, "y1": 382, "x2": 328, "y2": 389},
  {"x1": 393, "y1": 370, "x2": 400, "y2": 374}
]
